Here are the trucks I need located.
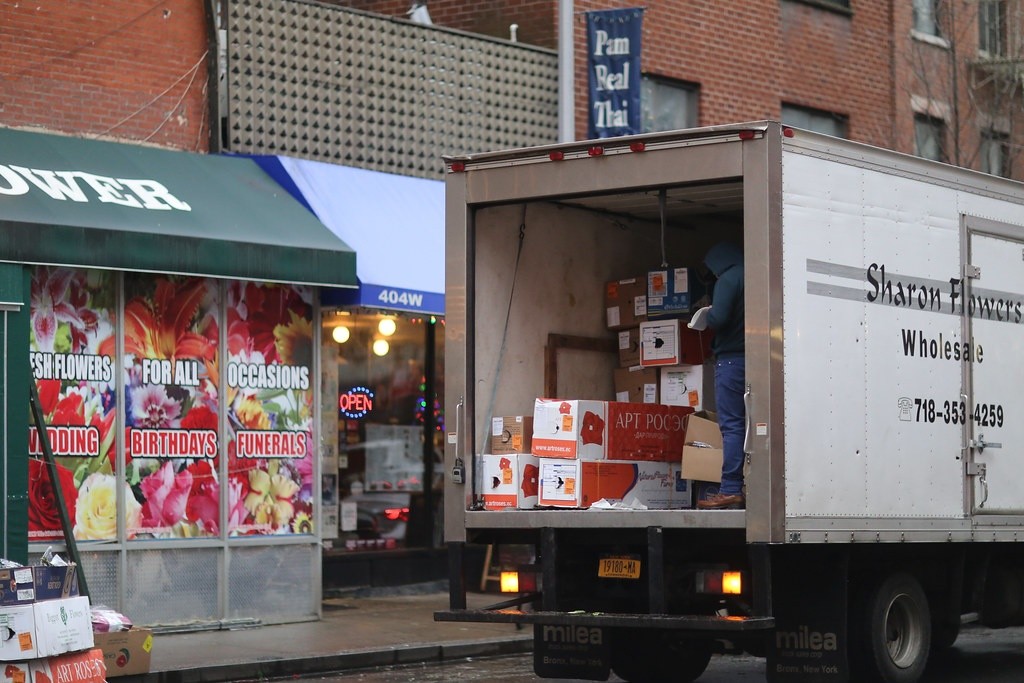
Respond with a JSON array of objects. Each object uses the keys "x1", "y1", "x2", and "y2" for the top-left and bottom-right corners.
[{"x1": 432, "y1": 121, "x2": 1024, "y2": 682}]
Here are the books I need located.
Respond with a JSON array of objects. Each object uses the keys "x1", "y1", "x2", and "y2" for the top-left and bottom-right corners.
[{"x1": 688, "y1": 306, "x2": 709, "y2": 331}]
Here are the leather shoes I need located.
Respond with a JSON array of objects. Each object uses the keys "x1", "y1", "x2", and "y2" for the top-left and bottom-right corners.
[{"x1": 697, "y1": 492, "x2": 744, "y2": 510}]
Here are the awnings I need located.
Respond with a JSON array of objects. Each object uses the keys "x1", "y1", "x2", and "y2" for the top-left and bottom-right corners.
[
  {"x1": 225, "y1": 153, "x2": 445, "y2": 316},
  {"x1": 0, "y1": 127, "x2": 361, "y2": 290}
]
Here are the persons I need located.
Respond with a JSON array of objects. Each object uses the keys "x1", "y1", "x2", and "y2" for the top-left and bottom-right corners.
[{"x1": 697, "y1": 243, "x2": 745, "y2": 509}]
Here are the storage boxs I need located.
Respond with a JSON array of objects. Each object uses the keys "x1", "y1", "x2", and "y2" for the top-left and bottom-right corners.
[
  {"x1": 0, "y1": 551, "x2": 153, "y2": 683},
  {"x1": 482, "y1": 263, "x2": 725, "y2": 508}
]
